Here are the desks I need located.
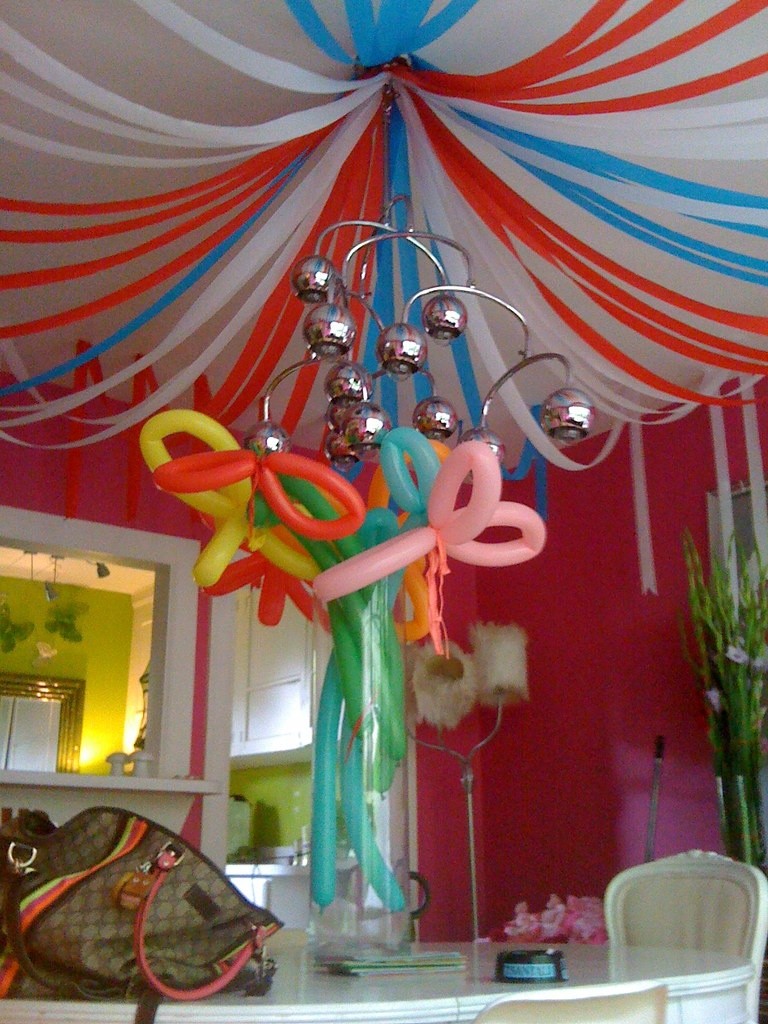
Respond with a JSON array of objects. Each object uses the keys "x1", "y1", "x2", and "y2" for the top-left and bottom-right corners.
[{"x1": 1, "y1": 927, "x2": 757, "y2": 1024}]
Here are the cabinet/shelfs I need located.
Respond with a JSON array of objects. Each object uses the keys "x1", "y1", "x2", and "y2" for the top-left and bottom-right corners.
[
  {"x1": 229, "y1": 876, "x2": 352, "y2": 928},
  {"x1": 230, "y1": 573, "x2": 369, "y2": 769}
]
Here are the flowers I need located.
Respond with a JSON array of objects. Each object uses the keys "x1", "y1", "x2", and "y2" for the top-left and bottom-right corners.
[
  {"x1": 676, "y1": 526, "x2": 768, "y2": 873},
  {"x1": 472, "y1": 893, "x2": 609, "y2": 945}
]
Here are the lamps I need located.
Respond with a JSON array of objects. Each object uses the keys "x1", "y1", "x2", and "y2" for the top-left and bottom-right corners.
[
  {"x1": 24, "y1": 551, "x2": 110, "y2": 602},
  {"x1": 241, "y1": 67, "x2": 595, "y2": 475},
  {"x1": 401, "y1": 619, "x2": 528, "y2": 942}
]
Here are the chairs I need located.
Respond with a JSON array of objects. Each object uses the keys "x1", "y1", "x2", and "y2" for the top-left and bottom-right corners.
[
  {"x1": 473, "y1": 980, "x2": 668, "y2": 1024},
  {"x1": 604, "y1": 850, "x2": 768, "y2": 1023}
]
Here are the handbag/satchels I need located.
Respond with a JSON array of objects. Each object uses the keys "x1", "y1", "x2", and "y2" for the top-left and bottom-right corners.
[{"x1": 0, "y1": 806, "x2": 284, "y2": 1024}]
